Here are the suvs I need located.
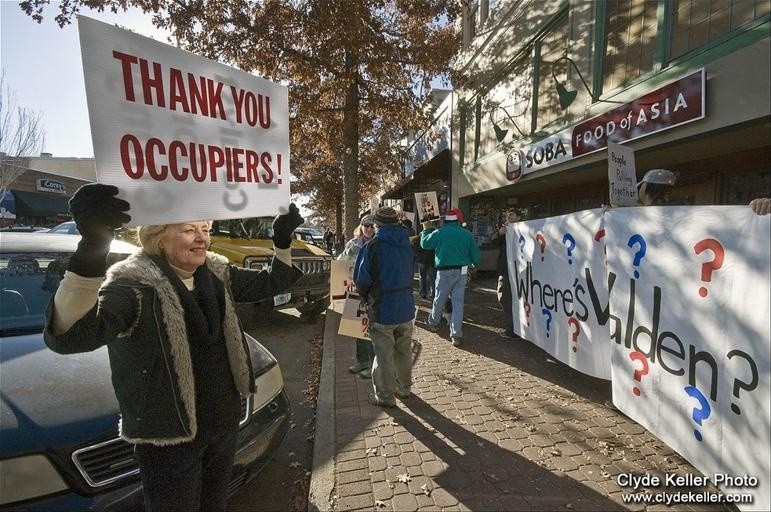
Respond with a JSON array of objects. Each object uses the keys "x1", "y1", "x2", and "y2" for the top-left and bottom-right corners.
[
  {"x1": 117, "y1": 209, "x2": 333, "y2": 326},
  {"x1": 0, "y1": 218, "x2": 78, "y2": 234},
  {"x1": 295, "y1": 225, "x2": 324, "y2": 244}
]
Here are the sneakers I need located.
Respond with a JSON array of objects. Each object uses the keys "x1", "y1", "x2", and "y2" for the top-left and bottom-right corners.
[
  {"x1": 368, "y1": 392, "x2": 397, "y2": 409},
  {"x1": 423, "y1": 316, "x2": 440, "y2": 333},
  {"x1": 396, "y1": 388, "x2": 412, "y2": 400},
  {"x1": 451, "y1": 336, "x2": 462, "y2": 346},
  {"x1": 499, "y1": 332, "x2": 522, "y2": 340},
  {"x1": 348, "y1": 363, "x2": 372, "y2": 379}
]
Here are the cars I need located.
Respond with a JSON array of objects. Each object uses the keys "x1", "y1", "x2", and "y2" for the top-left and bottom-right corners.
[{"x1": 0, "y1": 232, "x2": 294, "y2": 511}]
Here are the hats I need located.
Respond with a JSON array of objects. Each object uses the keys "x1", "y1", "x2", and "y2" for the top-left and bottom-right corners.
[
  {"x1": 360, "y1": 214, "x2": 374, "y2": 225},
  {"x1": 373, "y1": 206, "x2": 399, "y2": 226},
  {"x1": 444, "y1": 210, "x2": 467, "y2": 227},
  {"x1": 633, "y1": 169, "x2": 677, "y2": 188}
]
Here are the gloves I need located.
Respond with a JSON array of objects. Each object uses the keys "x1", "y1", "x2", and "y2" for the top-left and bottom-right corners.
[
  {"x1": 64, "y1": 183, "x2": 131, "y2": 279},
  {"x1": 271, "y1": 203, "x2": 305, "y2": 250}
]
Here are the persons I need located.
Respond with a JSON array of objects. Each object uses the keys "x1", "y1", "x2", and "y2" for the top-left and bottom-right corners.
[
  {"x1": 602, "y1": 168, "x2": 677, "y2": 205},
  {"x1": 42, "y1": 182, "x2": 305, "y2": 512},
  {"x1": 326, "y1": 231, "x2": 335, "y2": 260},
  {"x1": 415, "y1": 217, "x2": 439, "y2": 298},
  {"x1": 323, "y1": 227, "x2": 330, "y2": 244},
  {"x1": 401, "y1": 219, "x2": 416, "y2": 237},
  {"x1": 352, "y1": 206, "x2": 417, "y2": 408},
  {"x1": 336, "y1": 215, "x2": 376, "y2": 378},
  {"x1": 749, "y1": 197, "x2": 771, "y2": 216},
  {"x1": 490, "y1": 207, "x2": 528, "y2": 338},
  {"x1": 419, "y1": 208, "x2": 481, "y2": 346}
]
